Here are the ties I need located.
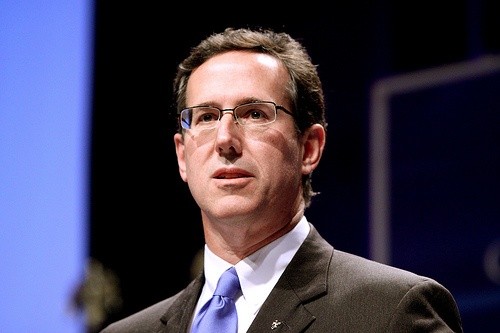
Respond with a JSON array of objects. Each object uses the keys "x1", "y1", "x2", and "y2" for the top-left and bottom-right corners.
[{"x1": 190, "y1": 266, "x2": 243, "y2": 333}]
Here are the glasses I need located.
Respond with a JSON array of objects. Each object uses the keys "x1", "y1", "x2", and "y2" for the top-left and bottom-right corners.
[{"x1": 177, "y1": 101, "x2": 307, "y2": 130}]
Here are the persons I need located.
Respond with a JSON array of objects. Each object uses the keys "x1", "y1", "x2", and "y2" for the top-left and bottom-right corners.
[{"x1": 95, "y1": 27, "x2": 463, "y2": 333}]
